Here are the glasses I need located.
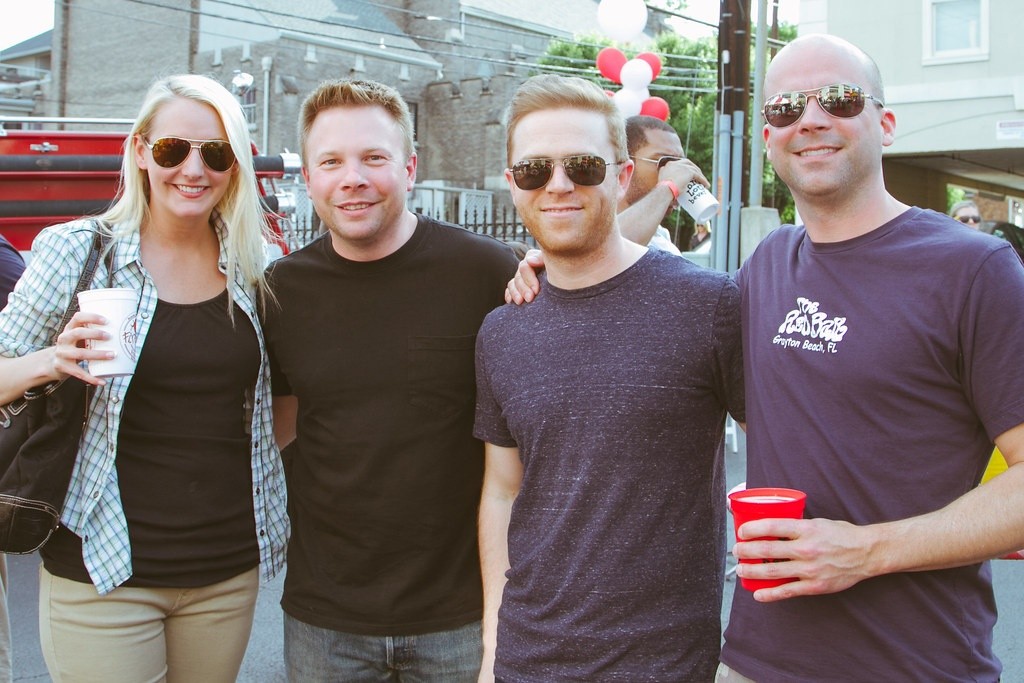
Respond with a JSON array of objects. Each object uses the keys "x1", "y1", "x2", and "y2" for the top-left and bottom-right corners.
[
  {"x1": 629, "y1": 156, "x2": 685, "y2": 174},
  {"x1": 761, "y1": 83, "x2": 885, "y2": 129},
  {"x1": 509, "y1": 155, "x2": 623, "y2": 191},
  {"x1": 142, "y1": 136, "x2": 236, "y2": 174},
  {"x1": 956, "y1": 215, "x2": 981, "y2": 223}
]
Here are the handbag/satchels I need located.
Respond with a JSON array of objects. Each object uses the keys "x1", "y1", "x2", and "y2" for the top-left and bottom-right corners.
[{"x1": 0, "y1": 214, "x2": 115, "y2": 555}]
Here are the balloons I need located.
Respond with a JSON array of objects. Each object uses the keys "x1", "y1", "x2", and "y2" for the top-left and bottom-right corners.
[
  {"x1": 613, "y1": 87, "x2": 650, "y2": 116},
  {"x1": 597, "y1": 47, "x2": 628, "y2": 85},
  {"x1": 598, "y1": 0, "x2": 648, "y2": 42},
  {"x1": 604, "y1": 90, "x2": 615, "y2": 97},
  {"x1": 620, "y1": 59, "x2": 653, "y2": 89},
  {"x1": 636, "y1": 52, "x2": 661, "y2": 80},
  {"x1": 641, "y1": 97, "x2": 669, "y2": 122}
]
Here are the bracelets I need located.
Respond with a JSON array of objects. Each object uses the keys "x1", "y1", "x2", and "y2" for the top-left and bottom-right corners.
[{"x1": 658, "y1": 180, "x2": 679, "y2": 200}]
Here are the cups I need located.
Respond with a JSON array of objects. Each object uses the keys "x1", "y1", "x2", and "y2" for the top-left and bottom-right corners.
[
  {"x1": 727, "y1": 487, "x2": 807, "y2": 592},
  {"x1": 676, "y1": 180, "x2": 720, "y2": 224},
  {"x1": 76, "y1": 288, "x2": 140, "y2": 378}
]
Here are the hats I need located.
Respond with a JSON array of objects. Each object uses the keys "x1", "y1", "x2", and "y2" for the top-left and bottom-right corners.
[{"x1": 1000, "y1": 223, "x2": 1024, "y2": 260}]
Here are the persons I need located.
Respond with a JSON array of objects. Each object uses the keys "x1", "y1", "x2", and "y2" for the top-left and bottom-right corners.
[
  {"x1": 0, "y1": 74, "x2": 292, "y2": 683},
  {"x1": 0, "y1": 234, "x2": 26, "y2": 683},
  {"x1": 505, "y1": 33, "x2": 1024, "y2": 683},
  {"x1": 950, "y1": 200, "x2": 981, "y2": 231},
  {"x1": 500, "y1": 241, "x2": 533, "y2": 261},
  {"x1": 473, "y1": 73, "x2": 746, "y2": 683},
  {"x1": 617, "y1": 115, "x2": 711, "y2": 257},
  {"x1": 253, "y1": 81, "x2": 522, "y2": 683}
]
